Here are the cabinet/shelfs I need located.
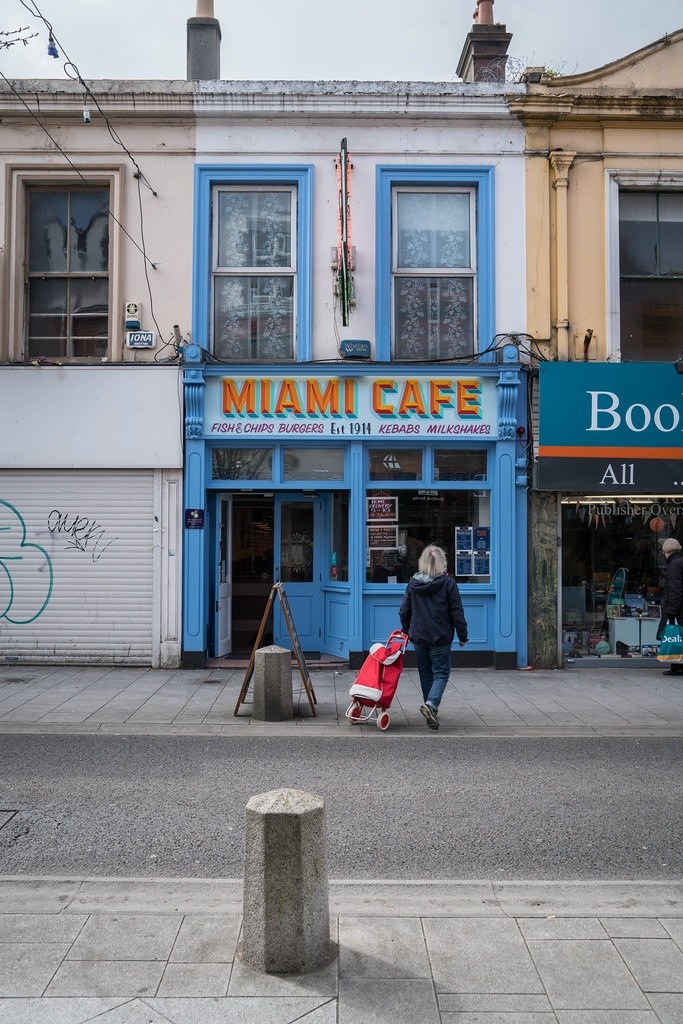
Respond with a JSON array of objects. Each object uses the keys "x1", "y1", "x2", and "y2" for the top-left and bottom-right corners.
[{"x1": 608, "y1": 617, "x2": 662, "y2": 654}]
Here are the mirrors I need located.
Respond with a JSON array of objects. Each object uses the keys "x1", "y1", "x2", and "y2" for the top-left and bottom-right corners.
[{"x1": 598, "y1": 567, "x2": 626, "y2": 643}]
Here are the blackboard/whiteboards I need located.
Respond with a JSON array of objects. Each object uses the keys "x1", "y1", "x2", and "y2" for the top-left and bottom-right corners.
[
  {"x1": 366, "y1": 496, "x2": 399, "y2": 522},
  {"x1": 276, "y1": 582, "x2": 313, "y2": 692},
  {"x1": 366, "y1": 526, "x2": 399, "y2": 550}
]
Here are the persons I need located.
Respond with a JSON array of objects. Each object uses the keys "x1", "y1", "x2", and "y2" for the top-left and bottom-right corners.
[
  {"x1": 656, "y1": 538, "x2": 683, "y2": 676},
  {"x1": 399, "y1": 544, "x2": 469, "y2": 731}
]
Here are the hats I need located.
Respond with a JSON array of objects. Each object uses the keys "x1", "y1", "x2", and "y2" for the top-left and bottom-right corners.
[{"x1": 662, "y1": 538, "x2": 682, "y2": 553}]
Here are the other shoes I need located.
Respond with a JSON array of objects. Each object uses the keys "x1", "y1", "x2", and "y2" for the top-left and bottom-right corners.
[
  {"x1": 663, "y1": 670, "x2": 683, "y2": 675},
  {"x1": 419, "y1": 703, "x2": 440, "y2": 731}
]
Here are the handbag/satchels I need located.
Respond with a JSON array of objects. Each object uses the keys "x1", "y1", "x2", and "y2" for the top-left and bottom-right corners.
[{"x1": 656, "y1": 616, "x2": 683, "y2": 663}]
